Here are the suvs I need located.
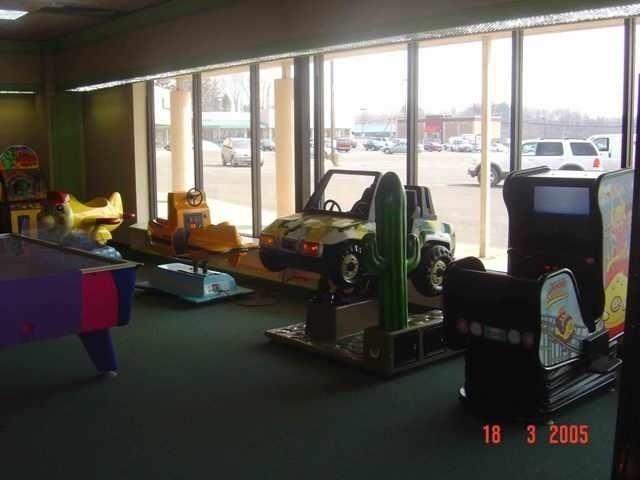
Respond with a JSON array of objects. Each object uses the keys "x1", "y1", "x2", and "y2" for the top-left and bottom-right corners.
[{"x1": 468, "y1": 136, "x2": 602, "y2": 187}]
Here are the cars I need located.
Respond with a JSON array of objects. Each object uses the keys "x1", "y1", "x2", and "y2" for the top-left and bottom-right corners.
[
  {"x1": 261, "y1": 139, "x2": 275, "y2": 151},
  {"x1": 328, "y1": 135, "x2": 503, "y2": 152},
  {"x1": 221, "y1": 137, "x2": 264, "y2": 166}
]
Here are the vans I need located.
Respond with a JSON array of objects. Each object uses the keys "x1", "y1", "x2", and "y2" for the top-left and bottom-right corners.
[{"x1": 588, "y1": 135, "x2": 636, "y2": 171}]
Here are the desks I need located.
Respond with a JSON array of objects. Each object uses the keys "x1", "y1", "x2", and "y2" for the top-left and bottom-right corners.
[{"x1": 0, "y1": 231, "x2": 144, "y2": 371}]
